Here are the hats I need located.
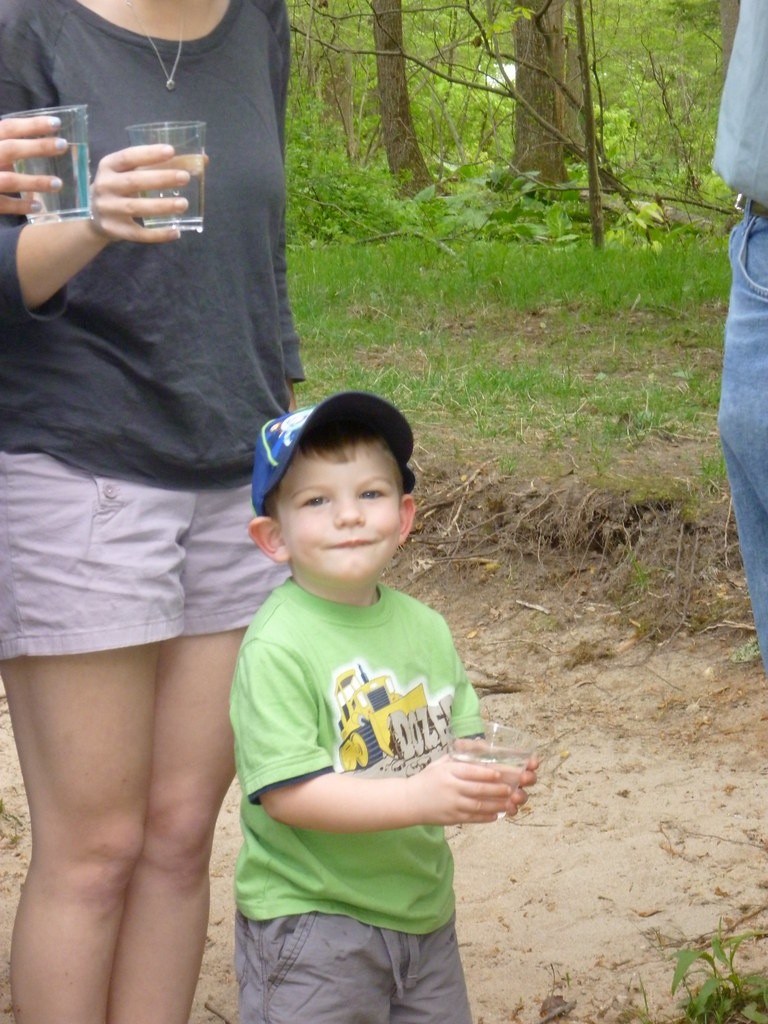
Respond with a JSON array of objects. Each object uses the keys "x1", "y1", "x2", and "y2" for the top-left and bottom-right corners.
[{"x1": 252, "y1": 392, "x2": 415, "y2": 516}]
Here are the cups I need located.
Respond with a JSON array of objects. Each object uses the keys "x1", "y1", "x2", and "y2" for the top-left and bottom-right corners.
[
  {"x1": 446, "y1": 719, "x2": 536, "y2": 819},
  {"x1": 0, "y1": 104, "x2": 93, "y2": 224},
  {"x1": 125, "y1": 121, "x2": 208, "y2": 231}
]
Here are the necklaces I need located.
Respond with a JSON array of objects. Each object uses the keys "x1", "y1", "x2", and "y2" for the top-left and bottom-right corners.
[{"x1": 127, "y1": 0, "x2": 185, "y2": 90}]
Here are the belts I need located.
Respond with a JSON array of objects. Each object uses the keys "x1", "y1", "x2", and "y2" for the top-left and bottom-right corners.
[{"x1": 738, "y1": 194, "x2": 768, "y2": 218}]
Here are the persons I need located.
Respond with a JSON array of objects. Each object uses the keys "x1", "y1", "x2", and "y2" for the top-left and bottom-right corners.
[
  {"x1": 712, "y1": 0, "x2": 768, "y2": 678},
  {"x1": 1, "y1": 0, "x2": 310, "y2": 1024},
  {"x1": 232, "y1": 391, "x2": 537, "y2": 1024}
]
